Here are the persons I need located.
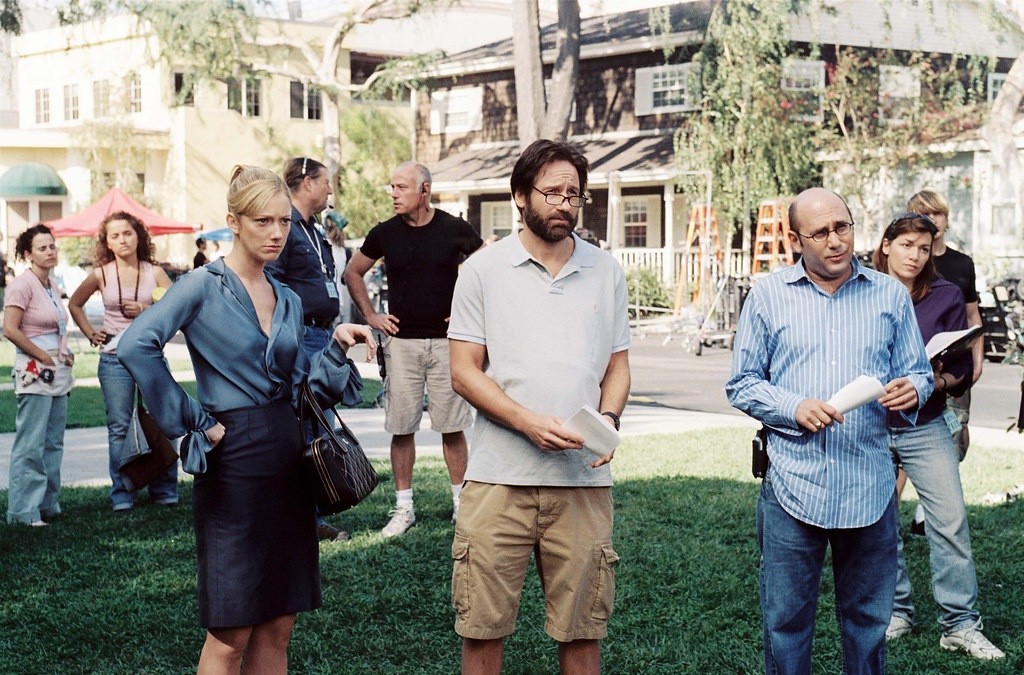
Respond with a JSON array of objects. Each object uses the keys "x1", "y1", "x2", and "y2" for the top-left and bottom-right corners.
[
  {"x1": 895, "y1": 191, "x2": 983, "y2": 539},
  {"x1": 449, "y1": 139, "x2": 632, "y2": 675},
  {"x1": 343, "y1": 162, "x2": 488, "y2": 540},
  {"x1": 67, "y1": 212, "x2": 178, "y2": 509},
  {"x1": 210, "y1": 242, "x2": 224, "y2": 260},
  {"x1": 117, "y1": 164, "x2": 377, "y2": 675},
  {"x1": 323, "y1": 227, "x2": 349, "y2": 324},
  {"x1": 0, "y1": 224, "x2": 75, "y2": 529},
  {"x1": 265, "y1": 157, "x2": 351, "y2": 543},
  {"x1": 726, "y1": 187, "x2": 934, "y2": 675},
  {"x1": 0, "y1": 253, "x2": 9, "y2": 313},
  {"x1": 195, "y1": 238, "x2": 210, "y2": 268},
  {"x1": 873, "y1": 214, "x2": 1003, "y2": 658}
]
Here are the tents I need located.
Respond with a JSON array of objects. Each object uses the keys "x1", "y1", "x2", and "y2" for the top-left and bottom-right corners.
[
  {"x1": 34, "y1": 188, "x2": 203, "y2": 238},
  {"x1": 200, "y1": 227, "x2": 233, "y2": 240}
]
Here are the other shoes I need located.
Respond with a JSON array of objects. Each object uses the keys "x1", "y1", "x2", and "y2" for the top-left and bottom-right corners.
[{"x1": 315, "y1": 524, "x2": 350, "y2": 543}]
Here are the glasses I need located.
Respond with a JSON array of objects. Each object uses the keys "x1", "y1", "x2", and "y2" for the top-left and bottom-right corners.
[
  {"x1": 889, "y1": 212, "x2": 931, "y2": 231},
  {"x1": 531, "y1": 185, "x2": 590, "y2": 207},
  {"x1": 797, "y1": 221, "x2": 855, "y2": 242}
]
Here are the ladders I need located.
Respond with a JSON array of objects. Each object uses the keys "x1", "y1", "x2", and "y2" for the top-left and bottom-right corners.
[
  {"x1": 751, "y1": 197, "x2": 794, "y2": 274},
  {"x1": 674, "y1": 201, "x2": 729, "y2": 316}
]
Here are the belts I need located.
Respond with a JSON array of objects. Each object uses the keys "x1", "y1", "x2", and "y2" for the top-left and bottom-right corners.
[{"x1": 305, "y1": 314, "x2": 337, "y2": 330}]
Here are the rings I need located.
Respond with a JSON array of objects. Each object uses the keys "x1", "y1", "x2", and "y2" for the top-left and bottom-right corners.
[{"x1": 816, "y1": 423, "x2": 821, "y2": 428}]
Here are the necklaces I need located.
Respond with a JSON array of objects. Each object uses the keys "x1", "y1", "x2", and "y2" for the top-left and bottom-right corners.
[{"x1": 116, "y1": 262, "x2": 139, "y2": 319}]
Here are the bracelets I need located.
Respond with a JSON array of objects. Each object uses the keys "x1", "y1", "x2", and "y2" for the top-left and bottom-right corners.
[
  {"x1": 139, "y1": 304, "x2": 143, "y2": 312},
  {"x1": 90, "y1": 333, "x2": 96, "y2": 343},
  {"x1": 940, "y1": 377, "x2": 947, "y2": 391}
]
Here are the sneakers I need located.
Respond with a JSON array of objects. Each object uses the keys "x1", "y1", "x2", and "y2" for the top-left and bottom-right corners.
[
  {"x1": 939, "y1": 617, "x2": 1005, "y2": 661},
  {"x1": 885, "y1": 614, "x2": 912, "y2": 641},
  {"x1": 373, "y1": 508, "x2": 416, "y2": 544}
]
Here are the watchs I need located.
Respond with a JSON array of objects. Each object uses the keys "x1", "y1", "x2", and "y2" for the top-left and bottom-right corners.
[{"x1": 602, "y1": 412, "x2": 620, "y2": 431}]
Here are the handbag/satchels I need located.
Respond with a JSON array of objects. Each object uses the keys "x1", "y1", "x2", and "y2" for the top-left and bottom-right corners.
[{"x1": 298, "y1": 375, "x2": 380, "y2": 516}]
